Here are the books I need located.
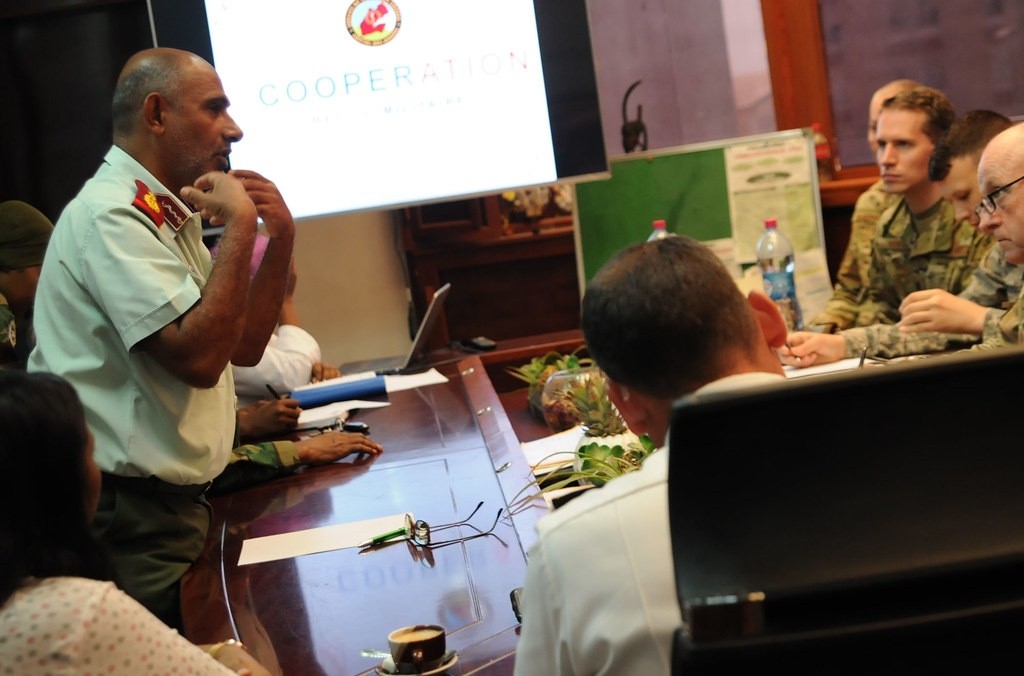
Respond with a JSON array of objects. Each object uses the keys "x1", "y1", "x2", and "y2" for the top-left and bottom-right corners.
[{"x1": 289, "y1": 370, "x2": 386, "y2": 429}]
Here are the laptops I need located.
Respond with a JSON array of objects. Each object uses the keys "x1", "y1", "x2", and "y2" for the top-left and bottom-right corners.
[{"x1": 339, "y1": 283, "x2": 451, "y2": 376}]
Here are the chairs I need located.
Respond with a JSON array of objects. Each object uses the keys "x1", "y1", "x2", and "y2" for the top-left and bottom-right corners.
[{"x1": 667, "y1": 341, "x2": 1024, "y2": 676}]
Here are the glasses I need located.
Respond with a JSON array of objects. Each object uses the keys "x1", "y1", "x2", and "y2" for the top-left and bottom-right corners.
[
  {"x1": 406, "y1": 524, "x2": 509, "y2": 567},
  {"x1": 404, "y1": 501, "x2": 503, "y2": 546},
  {"x1": 972, "y1": 176, "x2": 1024, "y2": 217}
]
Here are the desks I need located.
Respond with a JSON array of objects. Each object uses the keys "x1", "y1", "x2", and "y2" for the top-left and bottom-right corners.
[
  {"x1": 411, "y1": 176, "x2": 881, "y2": 362},
  {"x1": 178, "y1": 359, "x2": 526, "y2": 676}
]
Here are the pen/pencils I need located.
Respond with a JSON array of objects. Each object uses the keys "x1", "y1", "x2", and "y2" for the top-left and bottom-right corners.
[
  {"x1": 784, "y1": 342, "x2": 801, "y2": 362},
  {"x1": 859, "y1": 347, "x2": 866, "y2": 366},
  {"x1": 358, "y1": 527, "x2": 405, "y2": 547},
  {"x1": 266, "y1": 384, "x2": 280, "y2": 399}
]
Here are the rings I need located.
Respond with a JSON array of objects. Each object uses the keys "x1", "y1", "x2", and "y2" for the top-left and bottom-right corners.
[{"x1": 242, "y1": 177, "x2": 245, "y2": 181}]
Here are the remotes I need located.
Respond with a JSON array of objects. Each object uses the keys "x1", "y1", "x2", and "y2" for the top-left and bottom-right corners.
[{"x1": 472, "y1": 336, "x2": 495, "y2": 349}]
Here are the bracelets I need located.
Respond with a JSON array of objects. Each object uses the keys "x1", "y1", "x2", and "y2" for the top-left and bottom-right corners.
[{"x1": 209, "y1": 639, "x2": 243, "y2": 658}]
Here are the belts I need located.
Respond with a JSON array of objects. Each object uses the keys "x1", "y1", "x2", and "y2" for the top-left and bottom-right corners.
[{"x1": 101, "y1": 470, "x2": 213, "y2": 497}]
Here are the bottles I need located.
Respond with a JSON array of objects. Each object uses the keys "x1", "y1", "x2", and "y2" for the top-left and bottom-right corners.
[
  {"x1": 754, "y1": 218, "x2": 804, "y2": 332},
  {"x1": 646, "y1": 218, "x2": 669, "y2": 242}
]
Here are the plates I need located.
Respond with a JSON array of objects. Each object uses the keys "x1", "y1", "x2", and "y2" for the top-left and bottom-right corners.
[{"x1": 375, "y1": 649, "x2": 458, "y2": 676}]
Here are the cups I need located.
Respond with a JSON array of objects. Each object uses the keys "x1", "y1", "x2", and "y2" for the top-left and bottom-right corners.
[{"x1": 388, "y1": 623, "x2": 447, "y2": 674}]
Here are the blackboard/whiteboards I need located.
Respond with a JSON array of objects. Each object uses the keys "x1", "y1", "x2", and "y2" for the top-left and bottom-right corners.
[{"x1": 570, "y1": 127, "x2": 825, "y2": 304}]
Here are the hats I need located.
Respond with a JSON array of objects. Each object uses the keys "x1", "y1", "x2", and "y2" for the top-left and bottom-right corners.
[{"x1": 0, "y1": 200, "x2": 55, "y2": 268}]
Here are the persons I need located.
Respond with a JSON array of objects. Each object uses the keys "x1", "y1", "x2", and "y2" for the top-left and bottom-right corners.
[
  {"x1": 969, "y1": 121, "x2": 1024, "y2": 349},
  {"x1": 26, "y1": 49, "x2": 296, "y2": 600},
  {"x1": 777, "y1": 109, "x2": 1024, "y2": 369},
  {"x1": 206, "y1": 259, "x2": 383, "y2": 497},
  {"x1": 0, "y1": 201, "x2": 56, "y2": 363},
  {"x1": 514, "y1": 236, "x2": 786, "y2": 676},
  {"x1": 806, "y1": 79, "x2": 921, "y2": 332},
  {"x1": 0, "y1": 370, "x2": 274, "y2": 676}
]
